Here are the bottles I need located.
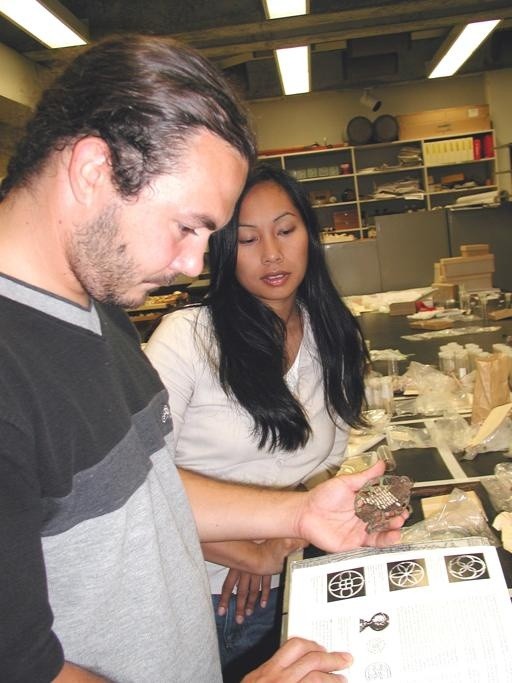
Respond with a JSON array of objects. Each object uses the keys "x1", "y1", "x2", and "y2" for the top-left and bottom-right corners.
[
  {"x1": 376, "y1": 444, "x2": 396, "y2": 471},
  {"x1": 362, "y1": 375, "x2": 397, "y2": 416},
  {"x1": 457, "y1": 283, "x2": 488, "y2": 321},
  {"x1": 503, "y1": 291, "x2": 512, "y2": 308},
  {"x1": 436, "y1": 339, "x2": 512, "y2": 380}
]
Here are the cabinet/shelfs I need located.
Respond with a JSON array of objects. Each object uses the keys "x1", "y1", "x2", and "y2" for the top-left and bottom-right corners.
[{"x1": 251, "y1": 128, "x2": 502, "y2": 248}]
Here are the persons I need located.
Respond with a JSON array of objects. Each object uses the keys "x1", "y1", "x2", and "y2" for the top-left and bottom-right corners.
[
  {"x1": 144, "y1": 163, "x2": 366, "y2": 682},
  {"x1": 1, "y1": 32, "x2": 412, "y2": 680}
]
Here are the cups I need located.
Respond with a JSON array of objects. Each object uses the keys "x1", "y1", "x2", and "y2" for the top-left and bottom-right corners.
[{"x1": 340, "y1": 162, "x2": 350, "y2": 174}]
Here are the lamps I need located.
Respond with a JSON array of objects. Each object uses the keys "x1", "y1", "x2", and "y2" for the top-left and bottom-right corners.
[
  {"x1": 420, "y1": 18, "x2": 498, "y2": 81},
  {"x1": 259, "y1": 0, "x2": 312, "y2": 96},
  {"x1": 1, "y1": 0, "x2": 93, "y2": 51}
]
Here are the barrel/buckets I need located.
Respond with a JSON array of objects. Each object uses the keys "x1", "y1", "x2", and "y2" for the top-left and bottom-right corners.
[
  {"x1": 373, "y1": 114, "x2": 398, "y2": 143},
  {"x1": 340, "y1": 116, "x2": 373, "y2": 145}
]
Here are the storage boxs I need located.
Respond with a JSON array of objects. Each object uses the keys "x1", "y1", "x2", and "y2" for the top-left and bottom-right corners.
[
  {"x1": 394, "y1": 100, "x2": 491, "y2": 140},
  {"x1": 427, "y1": 242, "x2": 496, "y2": 303}
]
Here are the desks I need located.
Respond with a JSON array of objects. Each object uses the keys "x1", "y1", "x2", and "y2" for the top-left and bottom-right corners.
[{"x1": 284, "y1": 276, "x2": 512, "y2": 683}]
